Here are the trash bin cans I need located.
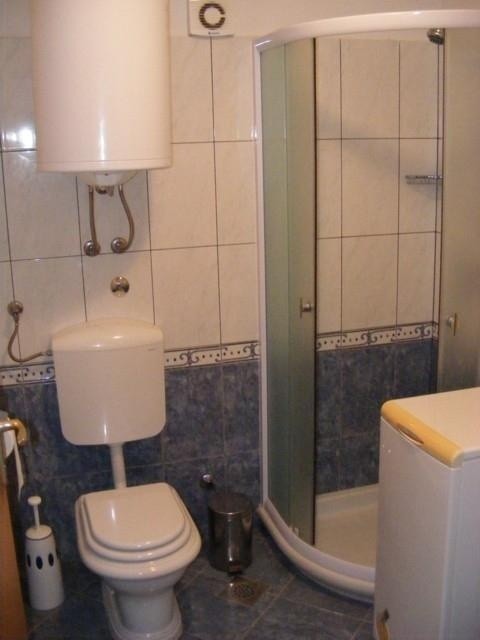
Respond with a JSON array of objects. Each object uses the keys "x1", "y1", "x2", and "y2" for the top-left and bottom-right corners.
[{"x1": 208, "y1": 493, "x2": 253, "y2": 573}]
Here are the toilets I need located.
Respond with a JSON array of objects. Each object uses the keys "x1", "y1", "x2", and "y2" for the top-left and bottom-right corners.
[{"x1": 50, "y1": 318, "x2": 203, "y2": 640}]
